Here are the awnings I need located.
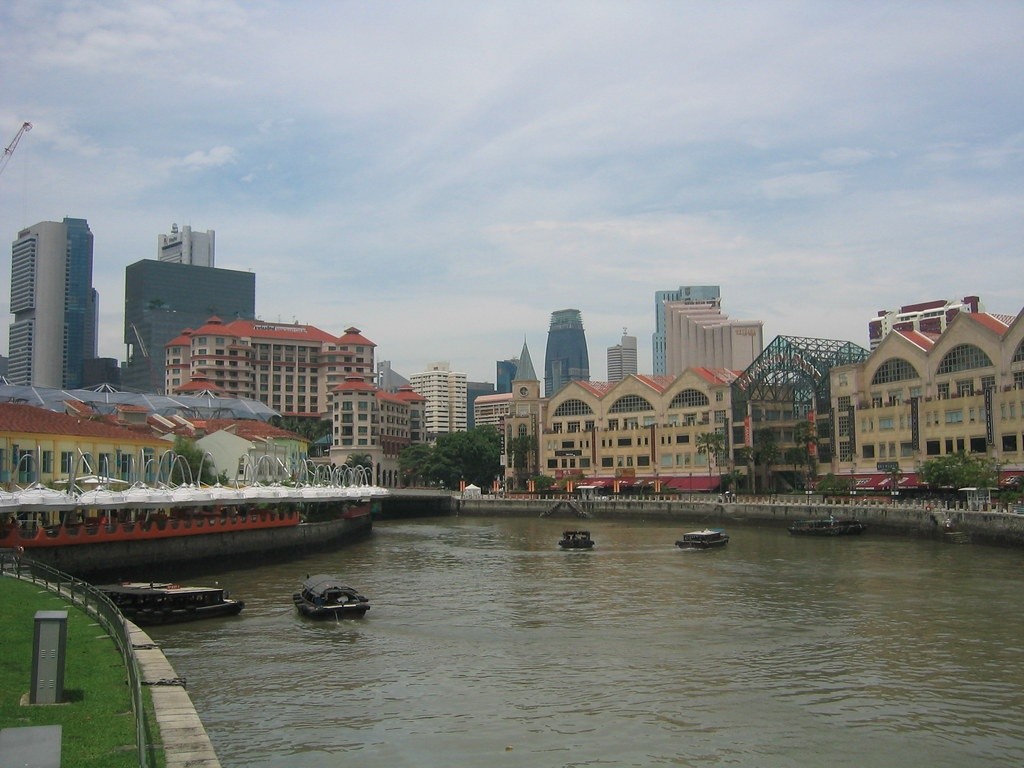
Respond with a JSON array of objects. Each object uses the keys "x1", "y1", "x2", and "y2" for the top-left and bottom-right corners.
[
  {"x1": 548, "y1": 476, "x2": 720, "y2": 490},
  {"x1": 804, "y1": 471, "x2": 1024, "y2": 489}
]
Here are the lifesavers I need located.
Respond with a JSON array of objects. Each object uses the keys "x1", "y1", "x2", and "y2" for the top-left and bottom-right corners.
[
  {"x1": 167, "y1": 584, "x2": 180, "y2": 589},
  {"x1": 926, "y1": 506, "x2": 931, "y2": 512}
]
